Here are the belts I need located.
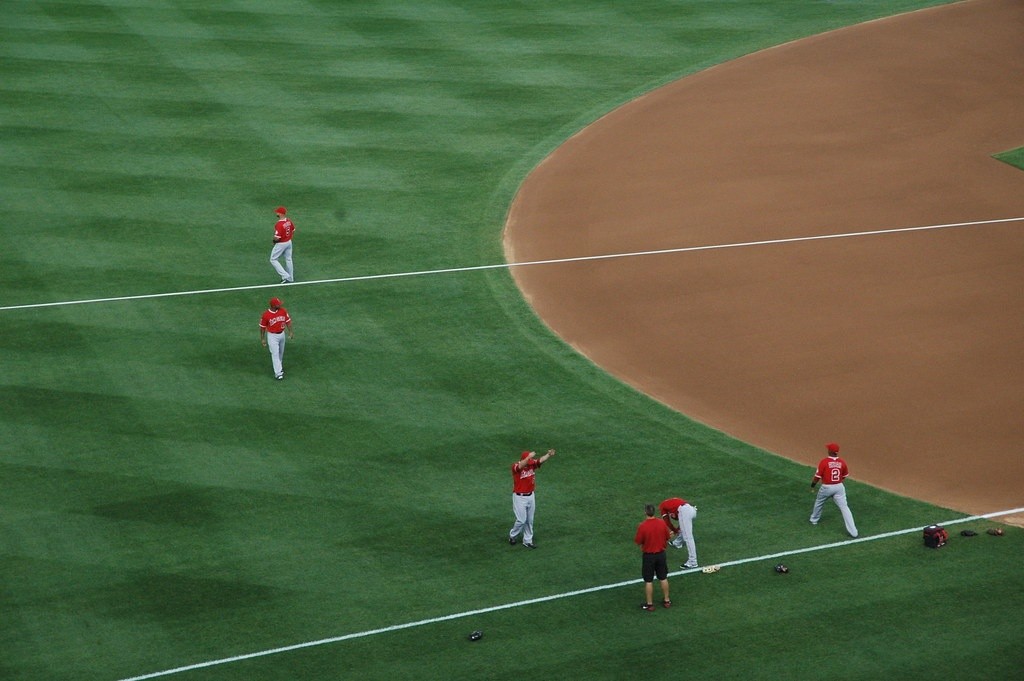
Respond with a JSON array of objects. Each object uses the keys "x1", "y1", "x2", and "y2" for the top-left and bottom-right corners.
[
  {"x1": 271, "y1": 331, "x2": 283, "y2": 334},
  {"x1": 517, "y1": 492, "x2": 532, "y2": 496},
  {"x1": 675, "y1": 502, "x2": 689, "y2": 514}
]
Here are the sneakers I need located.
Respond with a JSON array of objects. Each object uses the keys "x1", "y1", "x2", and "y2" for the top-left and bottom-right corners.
[
  {"x1": 680, "y1": 563, "x2": 698, "y2": 569},
  {"x1": 663, "y1": 598, "x2": 671, "y2": 608},
  {"x1": 640, "y1": 603, "x2": 654, "y2": 611},
  {"x1": 669, "y1": 540, "x2": 682, "y2": 549}
]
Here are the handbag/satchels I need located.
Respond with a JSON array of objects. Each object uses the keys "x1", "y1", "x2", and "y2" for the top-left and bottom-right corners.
[{"x1": 923, "y1": 525, "x2": 947, "y2": 548}]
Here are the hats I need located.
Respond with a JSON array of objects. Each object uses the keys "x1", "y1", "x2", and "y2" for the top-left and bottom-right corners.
[
  {"x1": 522, "y1": 451, "x2": 535, "y2": 465},
  {"x1": 274, "y1": 207, "x2": 286, "y2": 214},
  {"x1": 825, "y1": 443, "x2": 840, "y2": 454},
  {"x1": 270, "y1": 298, "x2": 283, "y2": 307}
]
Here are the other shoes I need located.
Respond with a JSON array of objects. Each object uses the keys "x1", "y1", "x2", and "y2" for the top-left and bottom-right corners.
[
  {"x1": 281, "y1": 280, "x2": 286, "y2": 283},
  {"x1": 523, "y1": 542, "x2": 536, "y2": 549},
  {"x1": 509, "y1": 537, "x2": 515, "y2": 544},
  {"x1": 277, "y1": 376, "x2": 283, "y2": 379}
]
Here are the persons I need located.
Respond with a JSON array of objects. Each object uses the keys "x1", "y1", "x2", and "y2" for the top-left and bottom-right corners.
[
  {"x1": 809, "y1": 442, "x2": 858, "y2": 538},
  {"x1": 658, "y1": 497, "x2": 698, "y2": 569},
  {"x1": 635, "y1": 505, "x2": 671, "y2": 611},
  {"x1": 269, "y1": 207, "x2": 295, "y2": 284},
  {"x1": 508, "y1": 449, "x2": 556, "y2": 549},
  {"x1": 258, "y1": 297, "x2": 294, "y2": 380}
]
